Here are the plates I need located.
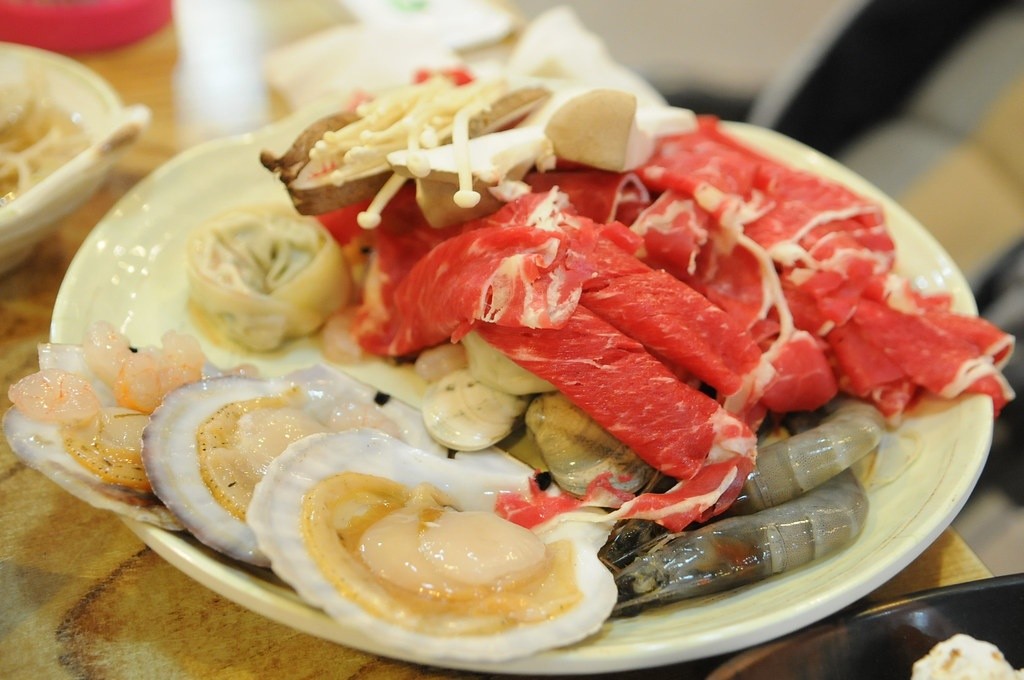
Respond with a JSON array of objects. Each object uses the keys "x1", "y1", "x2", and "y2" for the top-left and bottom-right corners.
[
  {"x1": 49, "y1": 83, "x2": 993, "y2": 674},
  {"x1": 704, "y1": 572, "x2": 1024, "y2": 680}
]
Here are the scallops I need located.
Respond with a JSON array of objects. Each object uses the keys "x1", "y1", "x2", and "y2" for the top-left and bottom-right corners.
[{"x1": 3, "y1": 344, "x2": 619, "y2": 662}]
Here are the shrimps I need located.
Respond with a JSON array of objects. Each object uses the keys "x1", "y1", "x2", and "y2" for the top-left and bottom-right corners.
[
  {"x1": 317, "y1": 301, "x2": 362, "y2": 366},
  {"x1": 414, "y1": 344, "x2": 465, "y2": 381},
  {"x1": 597, "y1": 401, "x2": 887, "y2": 627},
  {"x1": 7, "y1": 319, "x2": 208, "y2": 429}
]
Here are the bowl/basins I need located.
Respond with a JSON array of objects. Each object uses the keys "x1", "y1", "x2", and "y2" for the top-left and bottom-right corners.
[{"x1": 0, "y1": 41, "x2": 125, "y2": 277}]
